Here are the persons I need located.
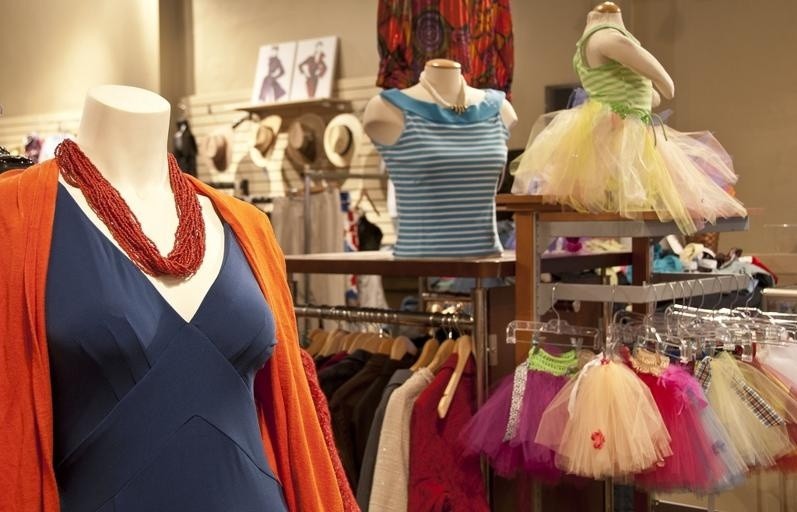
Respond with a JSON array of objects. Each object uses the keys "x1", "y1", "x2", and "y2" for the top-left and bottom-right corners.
[
  {"x1": 259, "y1": 45, "x2": 286, "y2": 105},
  {"x1": 362, "y1": 59, "x2": 519, "y2": 256},
  {"x1": 509, "y1": 3, "x2": 748, "y2": 237},
  {"x1": 297, "y1": 42, "x2": 327, "y2": 98},
  {"x1": 1, "y1": 81, "x2": 363, "y2": 512}
]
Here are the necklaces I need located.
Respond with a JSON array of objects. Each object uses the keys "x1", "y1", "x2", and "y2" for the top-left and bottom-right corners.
[
  {"x1": 54, "y1": 136, "x2": 206, "y2": 281},
  {"x1": 418, "y1": 72, "x2": 469, "y2": 114}
]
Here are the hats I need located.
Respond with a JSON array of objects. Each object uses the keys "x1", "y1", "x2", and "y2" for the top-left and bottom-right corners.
[{"x1": 172, "y1": 114, "x2": 363, "y2": 172}]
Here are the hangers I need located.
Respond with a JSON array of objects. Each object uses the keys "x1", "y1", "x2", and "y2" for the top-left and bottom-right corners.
[
  {"x1": 286, "y1": 304, "x2": 497, "y2": 420},
  {"x1": 507, "y1": 271, "x2": 795, "y2": 364}
]
